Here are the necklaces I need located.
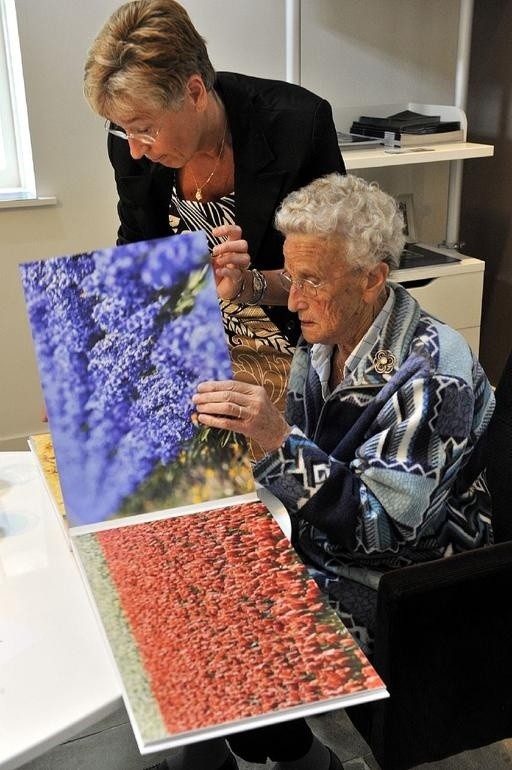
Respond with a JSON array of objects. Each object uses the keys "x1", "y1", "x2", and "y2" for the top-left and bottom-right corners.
[{"x1": 189, "y1": 123, "x2": 227, "y2": 200}]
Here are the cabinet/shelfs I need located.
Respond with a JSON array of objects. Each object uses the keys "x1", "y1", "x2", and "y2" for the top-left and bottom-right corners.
[{"x1": 340, "y1": 143, "x2": 495, "y2": 366}]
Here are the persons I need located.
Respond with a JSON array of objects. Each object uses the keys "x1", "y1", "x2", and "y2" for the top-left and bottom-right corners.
[
  {"x1": 193, "y1": 174, "x2": 496, "y2": 770},
  {"x1": 83, "y1": 0, "x2": 347, "y2": 355}
]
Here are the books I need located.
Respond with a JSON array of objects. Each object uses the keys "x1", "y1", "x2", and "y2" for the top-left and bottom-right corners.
[
  {"x1": 350, "y1": 109, "x2": 464, "y2": 148},
  {"x1": 18, "y1": 229, "x2": 391, "y2": 755}
]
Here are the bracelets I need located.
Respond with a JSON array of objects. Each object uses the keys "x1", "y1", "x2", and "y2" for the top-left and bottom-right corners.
[{"x1": 233, "y1": 267, "x2": 266, "y2": 307}]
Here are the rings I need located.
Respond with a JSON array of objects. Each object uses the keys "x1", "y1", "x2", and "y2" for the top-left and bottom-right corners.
[{"x1": 238, "y1": 406, "x2": 241, "y2": 418}]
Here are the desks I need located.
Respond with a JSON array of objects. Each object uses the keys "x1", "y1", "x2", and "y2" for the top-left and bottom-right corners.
[{"x1": 1, "y1": 450, "x2": 293, "y2": 770}]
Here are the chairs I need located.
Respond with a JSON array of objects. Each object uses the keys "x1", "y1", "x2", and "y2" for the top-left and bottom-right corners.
[{"x1": 345, "y1": 353, "x2": 512, "y2": 769}]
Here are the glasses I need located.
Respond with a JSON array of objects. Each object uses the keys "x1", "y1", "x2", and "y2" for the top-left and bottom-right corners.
[
  {"x1": 103, "y1": 113, "x2": 166, "y2": 146},
  {"x1": 276, "y1": 269, "x2": 366, "y2": 299}
]
